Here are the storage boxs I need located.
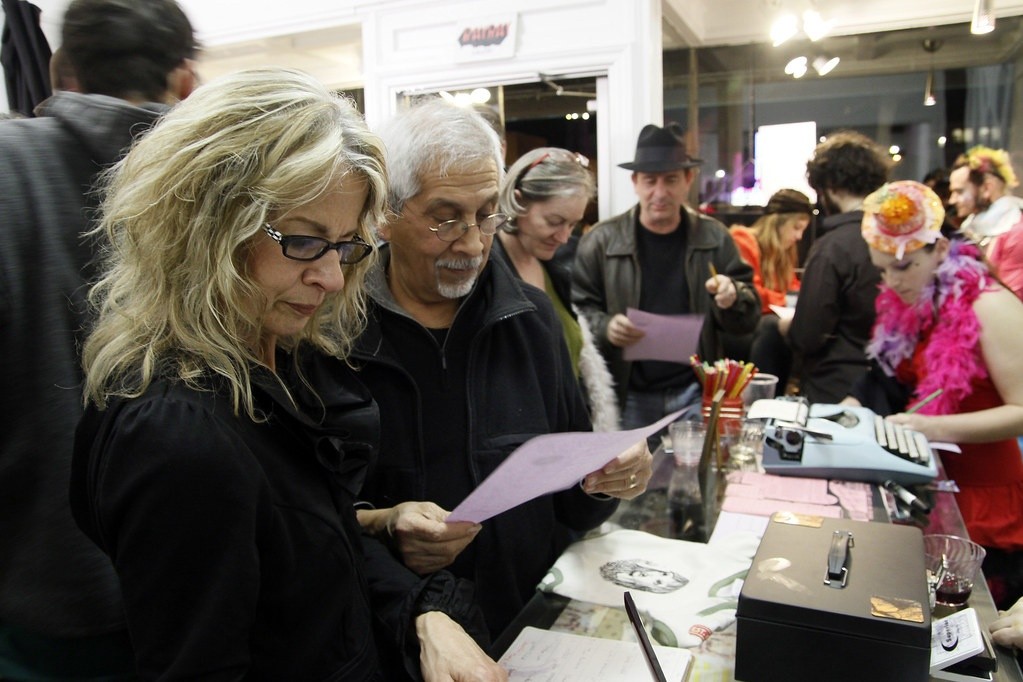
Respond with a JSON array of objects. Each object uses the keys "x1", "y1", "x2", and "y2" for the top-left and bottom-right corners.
[{"x1": 735, "y1": 511, "x2": 932, "y2": 682}]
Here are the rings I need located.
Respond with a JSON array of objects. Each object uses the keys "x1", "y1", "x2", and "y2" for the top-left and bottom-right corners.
[{"x1": 630, "y1": 474, "x2": 636, "y2": 488}]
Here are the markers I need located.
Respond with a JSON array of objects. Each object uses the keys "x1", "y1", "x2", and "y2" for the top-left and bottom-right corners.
[{"x1": 883, "y1": 479, "x2": 930, "y2": 514}]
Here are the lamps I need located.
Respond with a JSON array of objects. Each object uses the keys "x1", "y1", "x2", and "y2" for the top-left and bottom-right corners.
[
  {"x1": 813, "y1": 54, "x2": 840, "y2": 76},
  {"x1": 970, "y1": 0, "x2": 995, "y2": 35}
]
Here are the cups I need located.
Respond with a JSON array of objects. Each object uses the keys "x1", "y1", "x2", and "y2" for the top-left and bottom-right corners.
[
  {"x1": 724, "y1": 418, "x2": 764, "y2": 460},
  {"x1": 703, "y1": 397, "x2": 744, "y2": 436},
  {"x1": 924, "y1": 534, "x2": 986, "y2": 607},
  {"x1": 739, "y1": 373, "x2": 778, "y2": 410},
  {"x1": 670, "y1": 422, "x2": 708, "y2": 466}
]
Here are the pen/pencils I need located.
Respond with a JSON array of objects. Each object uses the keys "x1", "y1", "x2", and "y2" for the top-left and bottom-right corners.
[
  {"x1": 708, "y1": 261, "x2": 719, "y2": 286},
  {"x1": 907, "y1": 389, "x2": 943, "y2": 413},
  {"x1": 624, "y1": 591, "x2": 666, "y2": 682},
  {"x1": 690, "y1": 353, "x2": 759, "y2": 401}
]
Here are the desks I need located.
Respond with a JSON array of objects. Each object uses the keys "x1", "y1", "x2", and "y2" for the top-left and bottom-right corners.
[{"x1": 487, "y1": 421, "x2": 1023, "y2": 682}]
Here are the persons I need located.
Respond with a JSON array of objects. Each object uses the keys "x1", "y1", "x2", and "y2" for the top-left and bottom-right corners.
[
  {"x1": 350, "y1": 100, "x2": 653, "y2": 653},
  {"x1": 862, "y1": 181, "x2": 1023, "y2": 657},
  {"x1": 925, "y1": 149, "x2": 1023, "y2": 301},
  {"x1": 476, "y1": 104, "x2": 594, "y2": 387},
  {"x1": 0, "y1": 0, "x2": 199, "y2": 682},
  {"x1": 778, "y1": 133, "x2": 916, "y2": 415},
  {"x1": 726, "y1": 190, "x2": 813, "y2": 380},
  {"x1": 571, "y1": 124, "x2": 760, "y2": 453},
  {"x1": 66, "y1": 67, "x2": 508, "y2": 682}
]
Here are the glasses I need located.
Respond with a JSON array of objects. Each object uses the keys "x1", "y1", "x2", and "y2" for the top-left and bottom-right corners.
[
  {"x1": 220, "y1": 191, "x2": 374, "y2": 265},
  {"x1": 522, "y1": 151, "x2": 589, "y2": 176},
  {"x1": 403, "y1": 202, "x2": 512, "y2": 243}
]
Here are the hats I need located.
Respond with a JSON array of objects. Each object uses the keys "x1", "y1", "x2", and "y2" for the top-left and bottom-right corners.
[
  {"x1": 862, "y1": 180, "x2": 945, "y2": 260},
  {"x1": 949, "y1": 145, "x2": 1018, "y2": 190},
  {"x1": 617, "y1": 122, "x2": 704, "y2": 173}
]
